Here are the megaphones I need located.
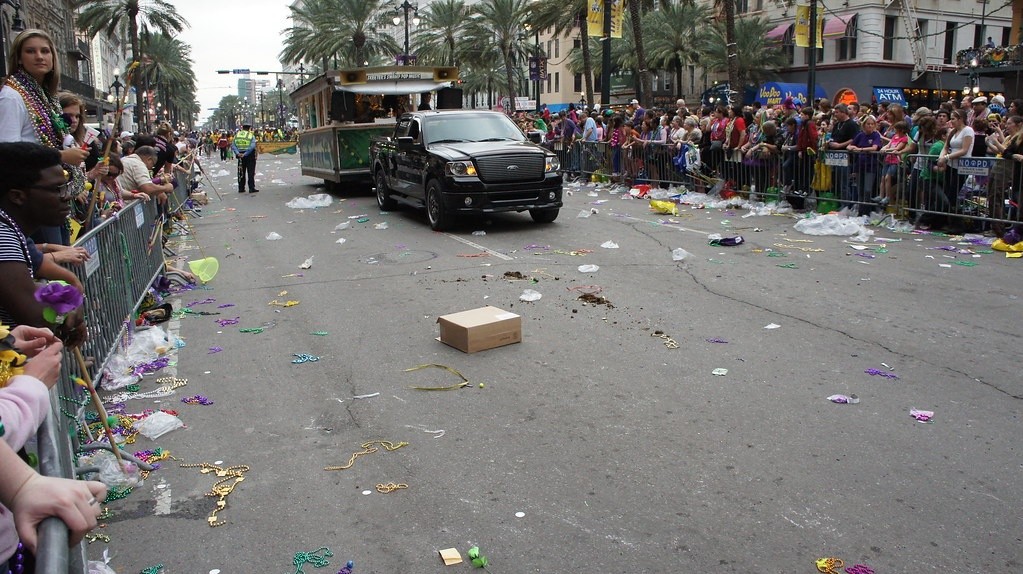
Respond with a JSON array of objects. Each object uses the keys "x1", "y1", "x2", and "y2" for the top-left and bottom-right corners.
[
  {"x1": 433, "y1": 66, "x2": 458, "y2": 81},
  {"x1": 339, "y1": 70, "x2": 367, "y2": 85}
]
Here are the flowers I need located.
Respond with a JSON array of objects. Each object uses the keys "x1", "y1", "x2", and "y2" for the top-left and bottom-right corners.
[{"x1": 31, "y1": 281, "x2": 85, "y2": 323}]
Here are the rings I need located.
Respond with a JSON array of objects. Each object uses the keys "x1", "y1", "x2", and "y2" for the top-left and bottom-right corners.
[
  {"x1": 89, "y1": 497, "x2": 97, "y2": 506},
  {"x1": 80, "y1": 253, "x2": 82, "y2": 257}
]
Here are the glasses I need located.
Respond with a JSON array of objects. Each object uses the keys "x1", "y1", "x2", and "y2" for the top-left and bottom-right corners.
[
  {"x1": 20, "y1": 182, "x2": 72, "y2": 198},
  {"x1": 107, "y1": 172, "x2": 119, "y2": 177}
]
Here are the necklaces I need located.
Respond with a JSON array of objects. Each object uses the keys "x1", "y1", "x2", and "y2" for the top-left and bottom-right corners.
[
  {"x1": 6, "y1": 70, "x2": 63, "y2": 148},
  {"x1": 651, "y1": 126, "x2": 661, "y2": 142},
  {"x1": 0, "y1": 209, "x2": 34, "y2": 278},
  {"x1": 61, "y1": 163, "x2": 85, "y2": 196},
  {"x1": 102, "y1": 179, "x2": 123, "y2": 204}
]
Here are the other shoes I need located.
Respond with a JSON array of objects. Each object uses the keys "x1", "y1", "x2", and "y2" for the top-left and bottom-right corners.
[
  {"x1": 239, "y1": 189, "x2": 246, "y2": 192},
  {"x1": 249, "y1": 188, "x2": 259, "y2": 193},
  {"x1": 879, "y1": 196, "x2": 889, "y2": 204},
  {"x1": 983, "y1": 230, "x2": 997, "y2": 237},
  {"x1": 871, "y1": 195, "x2": 884, "y2": 202}
]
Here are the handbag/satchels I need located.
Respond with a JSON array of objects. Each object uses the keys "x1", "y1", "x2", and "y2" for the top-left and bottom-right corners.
[
  {"x1": 810, "y1": 158, "x2": 832, "y2": 191},
  {"x1": 710, "y1": 140, "x2": 722, "y2": 151},
  {"x1": 685, "y1": 149, "x2": 701, "y2": 170}
]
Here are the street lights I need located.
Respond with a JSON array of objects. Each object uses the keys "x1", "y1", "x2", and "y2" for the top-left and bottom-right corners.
[
  {"x1": 578, "y1": 91, "x2": 588, "y2": 112},
  {"x1": 257, "y1": 91, "x2": 267, "y2": 142},
  {"x1": 274, "y1": 75, "x2": 287, "y2": 131},
  {"x1": 295, "y1": 62, "x2": 309, "y2": 85},
  {"x1": 108, "y1": 67, "x2": 129, "y2": 137},
  {"x1": 236, "y1": 95, "x2": 257, "y2": 131},
  {"x1": 393, "y1": 0, "x2": 420, "y2": 65}
]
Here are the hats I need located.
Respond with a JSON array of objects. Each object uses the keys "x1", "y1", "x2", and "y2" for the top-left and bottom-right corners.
[
  {"x1": 243, "y1": 123, "x2": 251, "y2": 127},
  {"x1": 991, "y1": 94, "x2": 1005, "y2": 107},
  {"x1": 605, "y1": 110, "x2": 615, "y2": 116},
  {"x1": 972, "y1": 96, "x2": 988, "y2": 104},
  {"x1": 916, "y1": 106, "x2": 932, "y2": 116},
  {"x1": 631, "y1": 99, "x2": 639, "y2": 105},
  {"x1": 120, "y1": 131, "x2": 134, "y2": 138}
]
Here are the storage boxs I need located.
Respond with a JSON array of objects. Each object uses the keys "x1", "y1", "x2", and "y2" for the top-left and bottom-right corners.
[
  {"x1": 434, "y1": 304, "x2": 522, "y2": 355},
  {"x1": 192, "y1": 190, "x2": 210, "y2": 205}
]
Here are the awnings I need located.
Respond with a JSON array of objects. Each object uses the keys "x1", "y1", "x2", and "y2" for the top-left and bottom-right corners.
[
  {"x1": 823, "y1": 13, "x2": 857, "y2": 40},
  {"x1": 764, "y1": 23, "x2": 793, "y2": 48},
  {"x1": 699, "y1": 80, "x2": 756, "y2": 106},
  {"x1": 792, "y1": 17, "x2": 824, "y2": 43},
  {"x1": 754, "y1": 81, "x2": 827, "y2": 106}
]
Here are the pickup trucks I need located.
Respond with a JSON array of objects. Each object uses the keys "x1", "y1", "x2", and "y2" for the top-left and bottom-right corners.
[{"x1": 368, "y1": 108, "x2": 563, "y2": 233}]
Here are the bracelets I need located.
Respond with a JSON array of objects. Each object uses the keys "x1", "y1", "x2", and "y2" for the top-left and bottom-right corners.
[
  {"x1": 861, "y1": 148, "x2": 863, "y2": 152},
  {"x1": 751, "y1": 147, "x2": 755, "y2": 151},
  {"x1": 944, "y1": 154, "x2": 949, "y2": 160},
  {"x1": 764, "y1": 143, "x2": 767, "y2": 146},
  {"x1": 50, "y1": 252, "x2": 56, "y2": 262},
  {"x1": 42, "y1": 243, "x2": 48, "y2": 252}
]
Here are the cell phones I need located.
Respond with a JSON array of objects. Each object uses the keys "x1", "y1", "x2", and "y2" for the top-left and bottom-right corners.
[{"x1": 993, "y1": 123, "x2": 998, "y2": 128}]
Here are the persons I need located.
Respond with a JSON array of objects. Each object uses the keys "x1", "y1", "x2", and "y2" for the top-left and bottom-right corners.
[
  {"x1": 419, "y1": 92, "x2": 432, "y2": 110},
  {"x1": 0, "y1": 29, "x2": 198, "y2": 574},
  {"x1": 199, "y1": 126, "x2": 300, "y2": 161},
  {"x1": 510, "y1": 96, "x2": 1023, "y2": 239},
  {"x1": 231, "y1": 123, "x2": 259, "y2": 193},
  {"x1": 984, "y1": 37, "x2": 995, "y2": 49}
]
